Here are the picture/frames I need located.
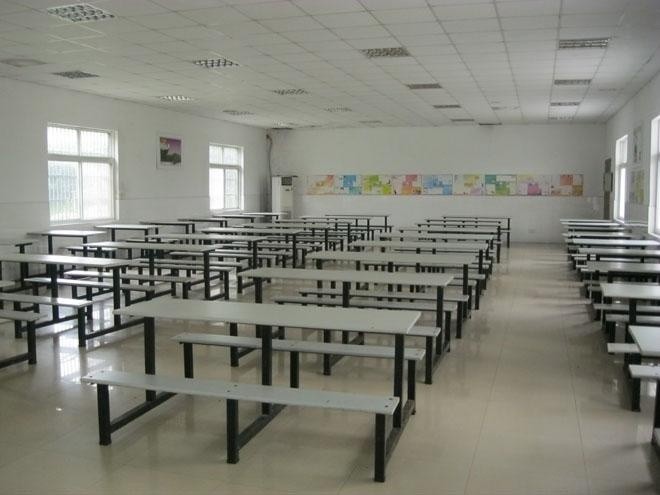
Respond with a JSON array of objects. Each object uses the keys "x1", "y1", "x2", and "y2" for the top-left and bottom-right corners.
[{"x1": 156, "y1": 133, "x2": 185, "y2": 172}]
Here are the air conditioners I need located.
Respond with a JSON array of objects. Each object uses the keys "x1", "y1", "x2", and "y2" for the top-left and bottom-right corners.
[{"x1": 272, "y1": 176, "x2": 295, "y2": 220}]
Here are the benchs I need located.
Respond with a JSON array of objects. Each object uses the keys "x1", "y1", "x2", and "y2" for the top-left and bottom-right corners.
[
  {"x1": 0, "y1": 213, "x2": 281, "y2": 373},
  {"x1": 223, "y1": 213, "x2": 518, "y2": 385},
  {"x1": 557, "y1": 213, "x2": 660, "y2": 453},
  {"x1": 86, "y1": 367, "x2": 401, "y2": 483},
  {"x1": 175, "y1": 330, "x2": 428, "y2": 422}
]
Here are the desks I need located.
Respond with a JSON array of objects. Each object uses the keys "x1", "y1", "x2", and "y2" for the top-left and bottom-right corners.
[{"x1": 111, "y1": 290, "x2": 422, "y2": 425}]
